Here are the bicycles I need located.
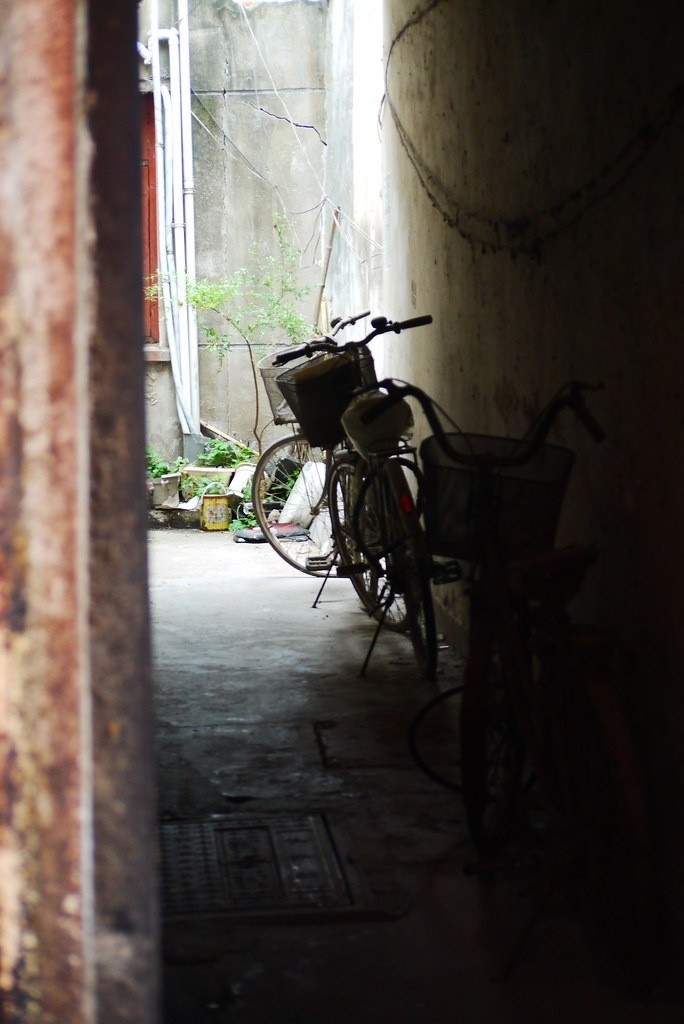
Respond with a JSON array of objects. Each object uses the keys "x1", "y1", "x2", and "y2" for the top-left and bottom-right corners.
[{"x1": 250, "y1": 307, "x2": 607, "y2": 888}]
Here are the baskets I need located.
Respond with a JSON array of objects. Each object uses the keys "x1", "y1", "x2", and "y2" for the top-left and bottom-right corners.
[
  {"x1": 420, "y1": 432, "x2": 574, "y2": 559},
  {"x1": 276, "y1": 346, "x2": 377, "y2": 448},
  {"x1": 258, "y1": 341, "x2": 325, "y2": 425}
]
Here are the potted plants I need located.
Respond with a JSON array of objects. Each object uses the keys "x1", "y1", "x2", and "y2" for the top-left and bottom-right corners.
[{"x1": 140, "y1": 437, "x2": 272, "y2": 511}]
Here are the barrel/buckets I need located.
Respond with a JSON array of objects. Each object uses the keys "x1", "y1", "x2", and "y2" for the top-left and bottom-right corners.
[
  {"x1": 198, "y1": 482, "x2": 230, "y2": 531},
  {"x1": 227, "y1": 461, "x2": 269, "y2": 507}
]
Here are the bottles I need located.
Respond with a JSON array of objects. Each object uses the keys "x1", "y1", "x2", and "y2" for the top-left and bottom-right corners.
[{"x1": 277, "y1": 460, "x2": 327, "y2": 529}]
